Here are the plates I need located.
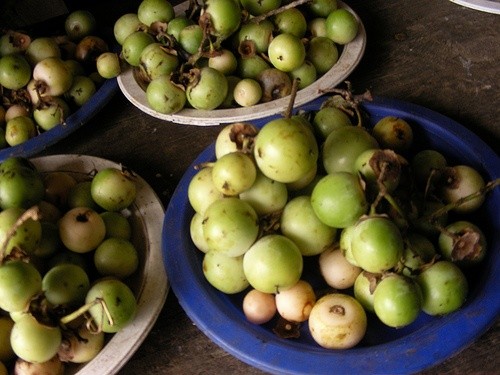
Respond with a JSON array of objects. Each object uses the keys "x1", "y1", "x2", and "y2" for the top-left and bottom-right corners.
[
  {"x1": 115, "y1": 1, "x2": 366, "y2": 127},
  {"x1": 0, "y1": 154, "x2": 168, "y2": 374},
  {"x1": 161, "y1": 94, "x2": 500, "y2": 375},
  {"x1": 0, "y1": 0, "x2": 119, "y2": 161}
]
